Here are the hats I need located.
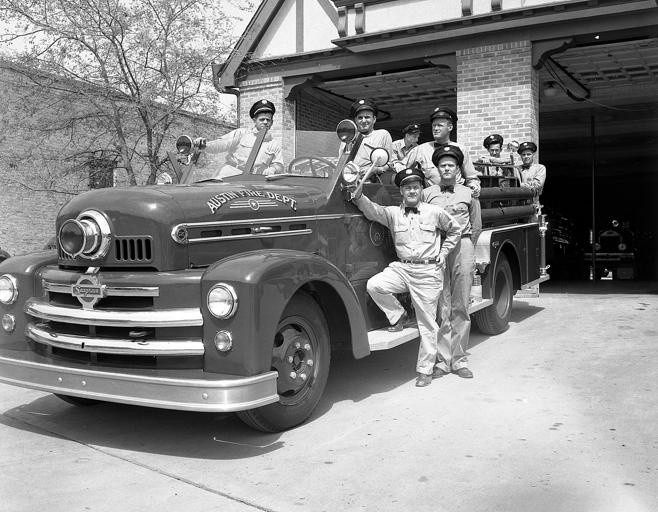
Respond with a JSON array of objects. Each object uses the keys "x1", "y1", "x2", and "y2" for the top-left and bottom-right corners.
[
  {"x1": 250, "y1": 99, "x2": 275, "y2": 118},
  {"x1": 352, "y1": 100, "x2": 536, "y2": 186}
]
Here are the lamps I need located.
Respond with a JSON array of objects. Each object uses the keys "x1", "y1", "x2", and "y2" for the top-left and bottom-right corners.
[{"x1": 541, "y1": 80, "x2": 564, "y2": 97}]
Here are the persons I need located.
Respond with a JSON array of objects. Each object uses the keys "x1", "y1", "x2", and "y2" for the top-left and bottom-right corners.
[
  {"x1": 471, "y1": 132, "x2": 515, "y2": 176},
  {"x1": 347, "y1": 168, "x2": 461, "y2": 387},
  {"x1": 193, "y1": 99, "x2": 284, "y2": 176},
  {"x1": 421, "y1": 145, "x2": 482, "y2": 379},
  {"x1": 394, "y1": 104, "x2": 482, "y2": 199},
  {"x1": 390, "y1": 122, "x2": 423, "y2": 166},
  {"x1": 513, "y1": 141, "x2": 547, "y2": 218},
  {"x1": 337, "y1": 97, "x2": 398, "y2": 181}
]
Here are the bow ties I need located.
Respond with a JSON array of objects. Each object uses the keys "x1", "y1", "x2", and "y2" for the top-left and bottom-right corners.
[
  {"x1": 404, "y1": 207, "x2": 417, "y2": 213},
  {"x1": 440, "y1": 184, "x2": 454, "y2": 193}
]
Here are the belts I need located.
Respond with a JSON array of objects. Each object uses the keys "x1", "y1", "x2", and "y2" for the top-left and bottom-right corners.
[
  {"x1": 226, "y1": 161, "x2": 245, "y2": 170},
  {"x1": 400, "y1": 259, "x2": 438, "y2": 264},
  {"x1": 440, "y1": 234, "x2": 469, "y2": 239}
]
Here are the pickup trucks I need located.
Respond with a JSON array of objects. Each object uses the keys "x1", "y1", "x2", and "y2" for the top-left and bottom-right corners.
[
  {"x1": 1, "y1": 118, "x2": 555, "y2": 434},
  {"x1": 583, "y1": 211, "x2": 644, "y2": 281}
]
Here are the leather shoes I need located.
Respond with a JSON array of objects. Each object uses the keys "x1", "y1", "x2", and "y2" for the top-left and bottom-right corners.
[
  {"x1": 416, "y1": 366, "x2": 473, "y2": 387},
  {"x1": 388, "y1": 311, "x2": 410, "y2": 332}
]
